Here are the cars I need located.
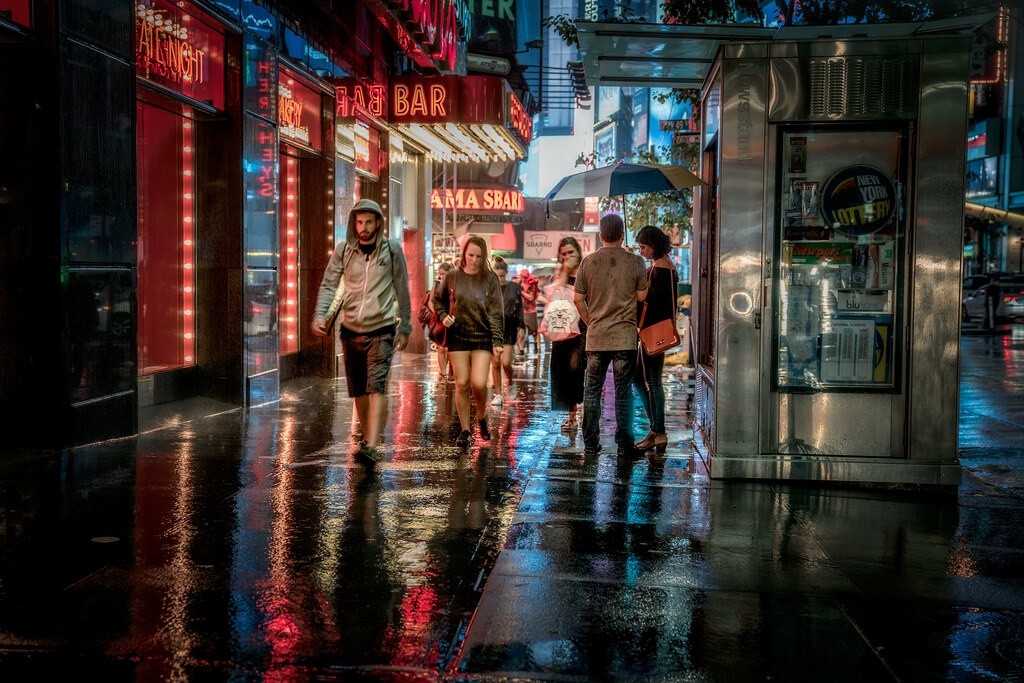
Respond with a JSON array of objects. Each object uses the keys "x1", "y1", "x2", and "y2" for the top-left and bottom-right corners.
[
  {"x1": 962, "y1": 275, "x2": 1024, "y2": 300},
  {"x1": 962, "y1": 283, "x2": 1024, "y2": 326},
  {"x1": 97, "y1": 281, "x2": 175, "y2": 337},
  {"x1": 244, "y1": 283, "x2": 280, "y2": 337}
]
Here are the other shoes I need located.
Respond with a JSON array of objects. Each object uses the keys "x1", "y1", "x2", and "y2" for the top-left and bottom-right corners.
[
  {"x1": 561, "y1": 419, "x2": 578, "y2": 431},
  {"x1": 477, "y1": 419, "x2": 491, "y2": 441},
  {"x1": 618, "y1": 443, "x2": 647, "y2": 457},
  {"x1": 586, "y1": 445, "x2": 602, "y2": 455},
  {"x1": 491, "y1": 394, "x2": 504, "y2": 404},
  {"x1": 455, "y1": 429, "x2": 472, "y2": 446},
  {"x1": 354, "y1": 446, "x2": 377, "y2": 463},
  {"x1": 504, "y1": 380, "x2": 517, "y2": 399}
]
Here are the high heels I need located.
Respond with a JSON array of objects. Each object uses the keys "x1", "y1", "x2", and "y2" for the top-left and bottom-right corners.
[{"x1": 636, "y1": 430, "x2": 667, "y2": 452}]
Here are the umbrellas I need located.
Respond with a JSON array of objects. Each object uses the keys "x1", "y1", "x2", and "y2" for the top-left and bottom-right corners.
[{"x1": 540, "y1": 159, "x2": 708, "y2": 246}]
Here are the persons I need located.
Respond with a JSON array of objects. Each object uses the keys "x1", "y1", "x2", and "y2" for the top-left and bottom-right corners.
[
  {"x1": 573, "y1": 213, "x2": 648, "y2": 455},
  {"x1": 310, "y1": 199, "x2": 413, "y2": 463},
  {"x1": 427, "y1": 263, "x2": 452, "y2": 376},
  {"x1": 634, "y1": 225, "x2": 679, "y2": 451},
  {"x1": 492, "y1": 255, "x2": 525, "y2": 405},
  {"x1": 550, "y1": 236, "x2": 587, "y2": 431},
  {"x1": 510, "y1": 268, "x2": 553, "y2": 354},
  {"x1": 433, "y1": 237, "x2": 504, "y2": 447}
]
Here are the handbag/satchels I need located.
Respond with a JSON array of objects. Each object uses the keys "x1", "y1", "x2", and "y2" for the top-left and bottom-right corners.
[
  {"x1": 538, "y1": 286, "x2": 581, "y2": 341},
  {"x1": 427, "y1": 313, "x2": 452, "y2": 348},
  {"x1": 418, "y1": 291, "x2": 433, "y2": 324},
  {"x1": 639, "y1": 318, "x2": 680, "y2": 355}
]
